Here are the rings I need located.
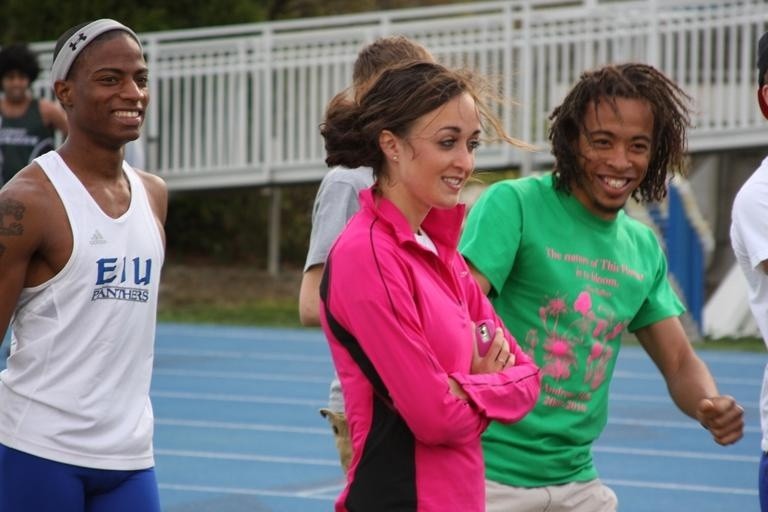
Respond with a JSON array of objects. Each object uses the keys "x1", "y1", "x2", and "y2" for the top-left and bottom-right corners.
[{"x1": 496, "y1": 357, "x2": 507, "y2": 367}]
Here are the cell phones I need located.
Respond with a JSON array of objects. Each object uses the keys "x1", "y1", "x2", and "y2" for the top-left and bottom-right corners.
[{"x1": 476, "y1": 319, "x2": 497, "y2": 358}]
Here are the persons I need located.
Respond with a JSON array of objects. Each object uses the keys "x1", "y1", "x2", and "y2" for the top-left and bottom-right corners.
[
  {"x1": 289, "y1": 32, "x2": 441, "y2": 480},
  {"x1": 448, "y1": 60, "x2": 747, "y2": 511},
  {"x1": 318, "y1": 55, "x2": 545, "y2": 511},
  {"x1": 0, "y1": 40, "x2": 70, "y2": 192},
  {"x1": 0, "y1": 15, "x2": 173, "y2": 512},
  {"x1": 723, "y1": 29, "x2": 768, "y2": 512}
]
